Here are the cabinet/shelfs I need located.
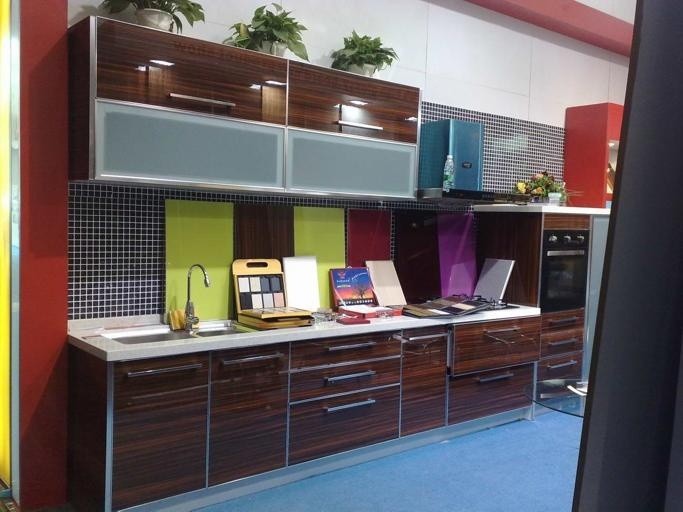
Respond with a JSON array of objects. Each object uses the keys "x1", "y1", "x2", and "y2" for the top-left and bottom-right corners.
[
  {"x1": 281, "y1": 53, "x2": 425, "y2": 207},
  {"x1": 564, "y1": 103, "x2": 625, "y2": 208},
  {"x1": 63, "y1": 15, "x2": 288, "y2": 199}
]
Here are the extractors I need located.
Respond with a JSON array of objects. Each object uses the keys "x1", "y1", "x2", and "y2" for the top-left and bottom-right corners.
[{"x1": 416, "y1": 118, "x2": 533, "y2": 203}]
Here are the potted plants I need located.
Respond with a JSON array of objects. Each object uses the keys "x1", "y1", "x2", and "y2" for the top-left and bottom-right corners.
[
  {"x1": 94, "y1": 1, "x2": 208, "y2": 40},
  {"x1": 327, "y1": 28, "x2": 402, "y2": 80},
  {"x1": 218, "y1": 2, "x2": 313, "y2": 65}
]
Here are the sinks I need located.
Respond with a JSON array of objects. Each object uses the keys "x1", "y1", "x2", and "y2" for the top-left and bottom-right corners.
[
  {"x1": 112, "y1": 331, "x2": 195, "y2": 345},
  {"x1": 190, "y1": 325, "x2": 249, "y2": 336}
]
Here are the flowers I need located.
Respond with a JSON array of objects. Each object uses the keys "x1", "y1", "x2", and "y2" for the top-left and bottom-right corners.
[{"x1": 509, "y1": 168, "x2": 583, "y2": 206}]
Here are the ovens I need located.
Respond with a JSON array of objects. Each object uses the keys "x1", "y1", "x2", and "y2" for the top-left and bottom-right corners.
[{"x1": 539, "y1": 229, "x2": 590, "y2": 313}]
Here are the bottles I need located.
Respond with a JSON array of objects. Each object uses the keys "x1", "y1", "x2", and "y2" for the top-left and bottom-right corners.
[{"x1": 442, "y1": 155, "x2": 454, "y2": 194}]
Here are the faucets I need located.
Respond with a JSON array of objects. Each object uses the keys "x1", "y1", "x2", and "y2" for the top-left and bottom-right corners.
[{"x1": 183, "y1": 264, "x2": 210, "y2": 327}]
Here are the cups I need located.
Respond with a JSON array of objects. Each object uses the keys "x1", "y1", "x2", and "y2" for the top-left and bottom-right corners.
[{"x1": 547, "y1": 192, "x2": 562, "y2": 205}]
